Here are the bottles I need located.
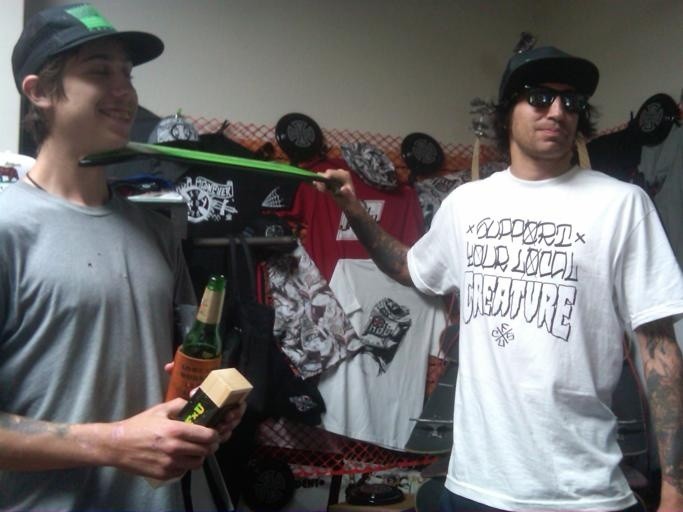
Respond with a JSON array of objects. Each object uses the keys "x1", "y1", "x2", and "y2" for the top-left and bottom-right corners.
[{"x1": 159, "y1": 271, "x2": 226, "y2": 420}]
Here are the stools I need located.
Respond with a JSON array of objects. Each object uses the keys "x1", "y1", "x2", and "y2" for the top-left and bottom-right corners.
[{"x1": 327, "y1": 493, "x2": 417, "y2": 512}]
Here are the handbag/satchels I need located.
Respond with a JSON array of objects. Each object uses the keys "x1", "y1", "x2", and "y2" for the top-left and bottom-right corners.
[{"x1": 212, "y1": 302, "x2": 326, "y2": 415}]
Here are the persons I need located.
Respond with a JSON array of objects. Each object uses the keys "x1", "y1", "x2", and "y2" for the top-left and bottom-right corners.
[
  {"x1": 0, "y1": 0, "x2": 249, "y2": 511},
  {"x1": 307, "y1": 44, "x2": 682, "y2": 510}
]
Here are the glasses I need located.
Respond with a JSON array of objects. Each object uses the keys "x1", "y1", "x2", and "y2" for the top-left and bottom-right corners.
[{"x1": 512, "y1": 83, "x2": 587, "y2": 111}]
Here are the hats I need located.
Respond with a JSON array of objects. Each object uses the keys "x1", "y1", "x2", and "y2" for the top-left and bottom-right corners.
[
  {"x1": 12, "y1": 4, "x2": 164, "y2": 92},
  {"x1": 251, "y1": 216, "x2": 296, "y2": 250},
  {"x1": 342, "y1": 143, "x2": 397, "y2": 189},
  {"x1": 148, "y1": 116, "x2": 199, "y2": 149},
  {"x1": 499, "y1": 47, "x2": 599, "y2": 104}
]
